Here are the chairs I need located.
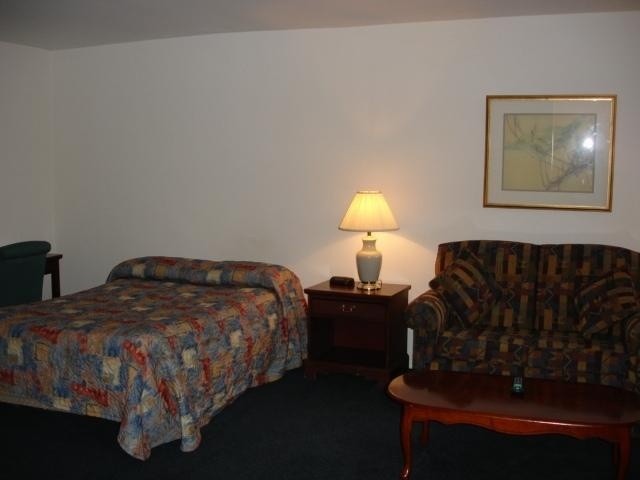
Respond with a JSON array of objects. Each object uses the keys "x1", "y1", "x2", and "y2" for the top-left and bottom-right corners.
[{"x1": 0, "y1": 240, "x2": 68, "y2": 307}]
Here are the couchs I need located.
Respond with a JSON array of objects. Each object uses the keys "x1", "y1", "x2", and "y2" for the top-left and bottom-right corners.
[{"x1": 400, "y1": 238, "x2": 639, "y2": 395}]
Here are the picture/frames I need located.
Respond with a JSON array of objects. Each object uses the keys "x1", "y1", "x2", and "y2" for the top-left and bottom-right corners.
[{"x1": 482, "y1": 94, "x2": 618, "y2": 213}]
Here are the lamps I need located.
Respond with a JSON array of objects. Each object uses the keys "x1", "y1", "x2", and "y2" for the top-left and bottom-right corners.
[{"x1": 337, "y1": 190, "x2": 401, "y2": 292}]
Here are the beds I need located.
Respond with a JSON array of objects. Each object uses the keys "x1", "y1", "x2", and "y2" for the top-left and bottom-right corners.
[{"x1": 1, "y1": 253, "x2": 314, "y2": 463}]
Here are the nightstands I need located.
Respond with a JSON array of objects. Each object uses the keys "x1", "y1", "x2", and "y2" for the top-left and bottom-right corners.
[{"x1": 298, "y1": 278, "x2": 413, "y2": 395}]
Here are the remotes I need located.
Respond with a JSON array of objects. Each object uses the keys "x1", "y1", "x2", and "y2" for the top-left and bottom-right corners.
[{"x1": 513, "y1": 377, "x2": 524, "y2": 393}]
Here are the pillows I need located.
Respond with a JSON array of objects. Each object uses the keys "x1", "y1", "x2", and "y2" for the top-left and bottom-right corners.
[
  {"x1": 427, "y1": 244, "x2": 517, "y2": 331},
  {"x1": 571, "y1": 259, "x2": 640, "y2": 341}
]
